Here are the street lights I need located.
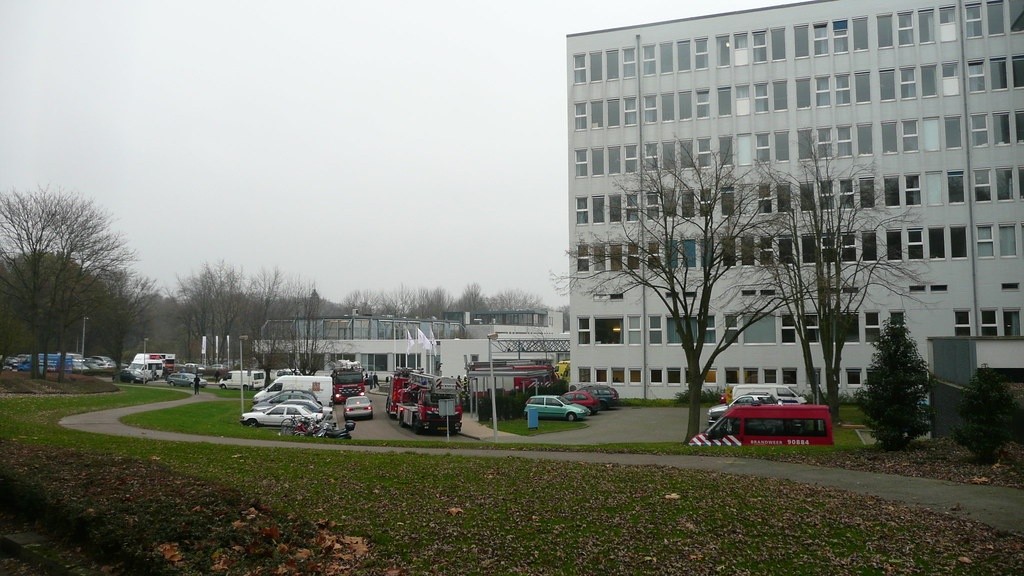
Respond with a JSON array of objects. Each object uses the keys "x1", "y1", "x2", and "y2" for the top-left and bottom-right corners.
[
  {"x1": 486, "y1": 333, "x2": 501, "y2": 443},
  {"x1": 238, "y1": 335, "x2": 248, "y2": 415},
  {"x1": 81, "y1": 317, "x2": 89, "y2": 374},
  {"x1": 143, "y1": 338, "x2": 148, "y2": 386}
]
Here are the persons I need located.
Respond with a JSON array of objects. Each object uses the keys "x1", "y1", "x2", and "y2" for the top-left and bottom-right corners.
[
  {"x1": 456, "y1": 375, "x2": 467, "y2": 392},
  {"x1": 365, "y1": 372, "x2": 380, "y2": 389},
  {"x1": 162, "y1": 367, "x2": 169, "y2": 380},
  {"x1": 194, "y1": 374, "x2": 200, "y2": 395},
  {"x1": 214, "y1": 371, "x2": 221, "y2": 383},
  {"x1": 153, "y1": 370, "x2": 157, "y2": 381}
]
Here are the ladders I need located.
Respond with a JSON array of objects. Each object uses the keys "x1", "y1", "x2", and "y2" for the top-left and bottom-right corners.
[{"x1": 408, "y1": 372, "x2": 434, "y2": 388}]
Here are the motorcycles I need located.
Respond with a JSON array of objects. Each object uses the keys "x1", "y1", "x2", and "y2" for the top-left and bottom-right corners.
[{"x1": 294, "y1": 412, "x2": 355, "y2": 440}]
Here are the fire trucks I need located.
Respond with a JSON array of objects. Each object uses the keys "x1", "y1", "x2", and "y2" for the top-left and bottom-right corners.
[{"x1": 386, "y1": 366, "x2": 465, "y2": 436}]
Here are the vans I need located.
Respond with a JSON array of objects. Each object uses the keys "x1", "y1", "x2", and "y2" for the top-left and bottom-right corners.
[
  {"x1": 127, "y1": 361, "x2": 163, "y2": 381},
  {"x1": 731, "y1": 384, "x2": 807, "y2": 406},
  {"x1": 688, "y1": 403, "x2": 836, "y2": 448},
  {"x1": 252, "y1": 375, "x2": 333, "y2": 407},
  {"x1": 219, "y1": 369, "x2": 303, "y2": 391}
]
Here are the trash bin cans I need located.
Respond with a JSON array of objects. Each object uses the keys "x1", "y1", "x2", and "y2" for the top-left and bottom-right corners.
[{"x1": 528, "y1": 408, "x2": 539, "y2": 428}]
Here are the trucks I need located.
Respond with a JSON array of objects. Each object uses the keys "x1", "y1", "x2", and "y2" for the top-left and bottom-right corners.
[
  {"x1": 331, "y1": 368, "x2": 367, "y2": 405},
  {"x1": 133, "y1": 353, "x2": 175, "y2": 366}
]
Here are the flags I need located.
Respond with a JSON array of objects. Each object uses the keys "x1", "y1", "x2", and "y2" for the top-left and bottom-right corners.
[
  {"x1": 227, "y1": 335, "x2": 229, "y2": 352},
  {"x1": 406, "y1": 328, "x2": 414, "y2": 355},
  {"x1": 216, "y1": 336, "x2": 218, "y2": 353},
  {"x1": 430, "y1": 326, "x2": 437, "y2": 357},
  {"x1": 416, "y1": 326, "x2": 432, "y2": 350},
  {"x1": 202, "y1": 336, "x2": 206, "y2": 354}
]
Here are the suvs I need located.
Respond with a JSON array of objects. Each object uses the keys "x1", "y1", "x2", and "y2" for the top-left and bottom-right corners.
[
  {"x1": 572, "y1": 385, "x2": 619, "y2": 411},
  {"x1": 166, "y1": 373, "x2": 208, "y2": 389},
  {"x1": 707, "y1": 391, "x2": 783, "y2": 419}
]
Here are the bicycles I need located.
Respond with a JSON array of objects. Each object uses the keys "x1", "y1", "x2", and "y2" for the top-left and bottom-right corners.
[{"x1": 280, "y1": 416, "x2": 297, "y2": 436}]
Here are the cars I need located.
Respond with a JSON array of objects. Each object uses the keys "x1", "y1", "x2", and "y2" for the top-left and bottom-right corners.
[
  {"x1": 1, "y1": 352, "x2": 128, "y2": 374},
  {"x1": 112, "y1": 367, "x2": 150, "y2": 384},
  {"x1": 523, "y1": 395, "x2": 592, "y2": 422},
  {"x1": 343, "y1": 396, "x2": 374, "y2": 420},
  {"x1": 175, "y1": 362, "x2": 223, "y2": 372},
  {"x1": 240, "y1": 404, "x2": 324, "y2": 429},
  {"x1": 551, "y1": 390, "x2": 601, "y2": 414},
  {"x1": 249, "y1": 389, "x2": 333, "y2": 419}
]
